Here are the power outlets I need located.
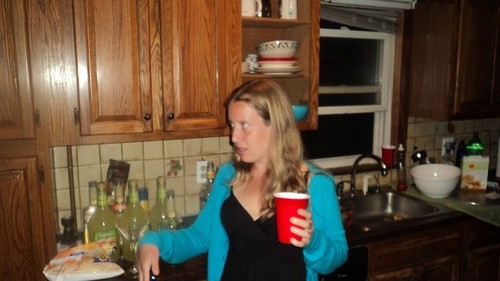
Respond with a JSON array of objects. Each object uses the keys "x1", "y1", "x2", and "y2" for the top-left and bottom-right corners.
[
  {"x1": 196, "y1": 160, "x2": 209, "y2": 183},
  {"x1": 442, "y1": 137, "x2": 454, "y2": 156}
]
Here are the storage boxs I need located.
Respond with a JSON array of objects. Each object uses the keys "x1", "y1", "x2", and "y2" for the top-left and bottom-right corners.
[{"x1": 460, "y1": 154, "x2": 489, "y2": 192}]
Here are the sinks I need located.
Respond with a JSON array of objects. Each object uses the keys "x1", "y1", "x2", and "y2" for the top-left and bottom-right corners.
[{"x1": 337, "y1": 188, "x2": 463, "y2": 236}]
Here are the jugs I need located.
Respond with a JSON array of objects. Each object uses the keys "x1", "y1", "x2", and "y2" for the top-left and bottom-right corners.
[
  {"x1": 241, "y1": 0, "x2": 262, "y2": 17},
  {"x1": 279, "y1": 0, "x2": 298, "y2": 19}
]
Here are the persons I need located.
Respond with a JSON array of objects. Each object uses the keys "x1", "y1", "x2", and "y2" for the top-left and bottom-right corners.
[{"x1": 138, "y1": 80, "x2": 348, "y2": 281}]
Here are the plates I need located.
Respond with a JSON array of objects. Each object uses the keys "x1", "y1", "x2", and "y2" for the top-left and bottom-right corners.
[{"x1": 251, "y1": 56, "x2": 303, "y2": 75}]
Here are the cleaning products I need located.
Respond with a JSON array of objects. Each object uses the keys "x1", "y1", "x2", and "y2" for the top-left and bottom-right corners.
[{"x1": 396, "y1": 143, "x2": 408, "y2": 191}]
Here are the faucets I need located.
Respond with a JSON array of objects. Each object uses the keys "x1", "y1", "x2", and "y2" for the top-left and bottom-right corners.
[{"x1": 348, "y1": 153, "x2": 388, "y2": 197}]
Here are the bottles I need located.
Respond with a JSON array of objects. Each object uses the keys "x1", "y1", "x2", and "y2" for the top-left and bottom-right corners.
[
  {"x1": 199, "y1": 161, "x2": 215, "y2": 212},
  {"x1": 397, "y1": 144, "x2": 407, "y2": 191},
  {"x1": 411, "y1": 150, "x2": 430, "y2": 166},
  {"x1": 263, "y1": 0, "x2": 271, "y2": 17},
  {"x1": 438, "y1": 142, "x2": 456, "y2": 166},
  {"x1": 83, "y1": 177, "x2": 184, "y2": 258}
]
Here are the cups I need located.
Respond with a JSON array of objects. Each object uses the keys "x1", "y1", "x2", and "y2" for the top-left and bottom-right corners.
[
  {"x1": 381, "y1": 144, "x2": 397, "y2": 167},
  {"x1": 273, "y1": 192, "x2": 309, "y2": 243}
]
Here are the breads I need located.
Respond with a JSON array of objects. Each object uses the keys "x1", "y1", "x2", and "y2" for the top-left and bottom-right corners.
[{"x1": 60, "y1": 255, "x2": 95, "y2": 274}]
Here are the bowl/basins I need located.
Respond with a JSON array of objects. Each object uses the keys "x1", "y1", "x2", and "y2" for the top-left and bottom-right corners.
[
  {"x1": 410, "y1": 164, "x2": 461, "y2": 198},
  {"x1": 257, "y1": 40, "x2": 299, "y2": 57}
]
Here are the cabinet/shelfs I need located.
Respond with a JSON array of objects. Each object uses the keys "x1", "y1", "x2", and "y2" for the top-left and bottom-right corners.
[
  {"x1": 406, "y1": 0, "x2": 500, "y2": 122},
  {"x1": 365, "y1": 217, "x2": 500, "y2": 281},
  {"x1": 0, "y1": 0, "x2": 321, "y2": 281}
]
